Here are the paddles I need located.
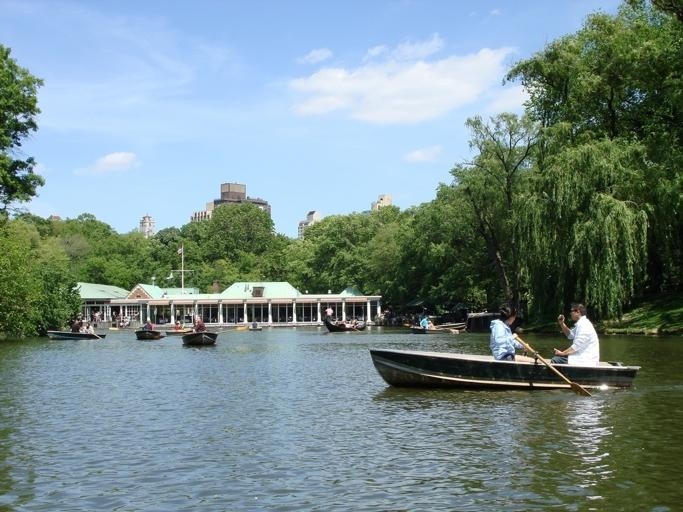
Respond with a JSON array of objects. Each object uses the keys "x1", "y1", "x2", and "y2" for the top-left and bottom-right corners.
[{"x1": 516, "y1": 337, "x2": 591, "y2": 397}]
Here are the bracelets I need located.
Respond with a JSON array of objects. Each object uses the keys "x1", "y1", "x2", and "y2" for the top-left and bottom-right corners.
[{"x1": 562, "y1": 324, "x2": 565, "y2": 329}]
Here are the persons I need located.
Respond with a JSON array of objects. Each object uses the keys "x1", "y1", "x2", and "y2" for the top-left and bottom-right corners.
[
  {"x1": 191, "y1": 319, "x2": 207, "y2": 333},
  {"x1": 352, "y1": 317, "x2": 358, "y2": 325},
  {"x1": 489, "y1": 306, "x2": 528, "y2": 361},
  {"x1": 141, "y1": 320, "x2": 152, "y2": 331},
  {"x1": 402, "y1": 312, "x2": 432, "y2": 330},
  {"x1": 252, "y1": 321, "x2": 257, "y2": 329},
  {"x1": 71, "y1": 310, "x2": 130, "y2": 334},
  {"x1": 549, "y1": 302, "x2": 600, "y2": 367},
  {"x1": 325, "y1": 305, "x2": 333, "y2": 320},
  {"x1": 176, "y1": 321, "x2": 181, "y2": 330}
]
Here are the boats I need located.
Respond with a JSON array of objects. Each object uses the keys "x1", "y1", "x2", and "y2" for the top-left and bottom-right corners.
[
  {"x1": 403, "y1": 322, "x2": 466, "y2": 333},
  {"x1": 108, "y1": 327, "x2": 129, "y2": 331},
  {"x1": 368, "y1": 345, "x2": 641, "y2": 393},
  {"x1": 181, "y1": 332, "x2": 220, "y2": 345},
  {"x1": 165, "y1": 328, "x2": 192, "y2": 337},
  {"x1": 248, "y1": 326, "x2": 262, "y2": 331},
  {"x1": 135, "y1": 330, "x2": 162, "y2": 340},
  {"x1": 323, "y1": 319, "x2": 366, "y2": 332},
  {"x1": 46, "y1": 329, "x2": 107, "y2": 341},
  {"x1": 236, "y1": 326, "x2": 247, "y2": 330}
]
